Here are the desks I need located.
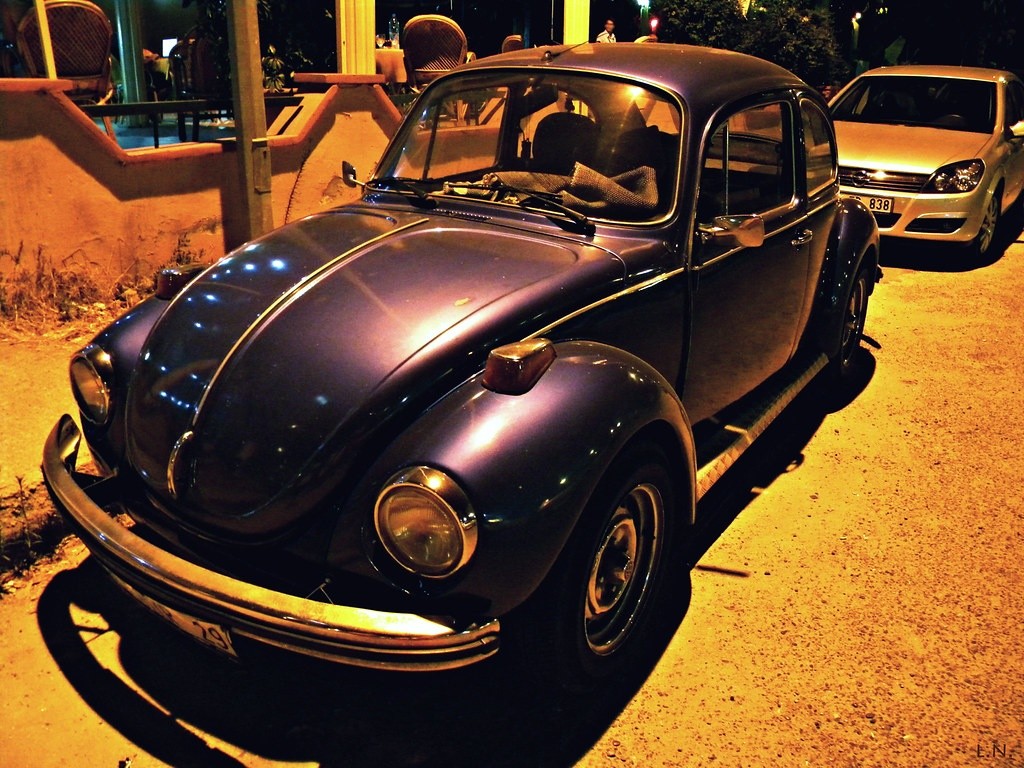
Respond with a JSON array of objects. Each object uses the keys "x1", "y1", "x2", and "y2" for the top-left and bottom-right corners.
[{"x1": 376, "y1": 48, "x2": 408, "y2": 94}]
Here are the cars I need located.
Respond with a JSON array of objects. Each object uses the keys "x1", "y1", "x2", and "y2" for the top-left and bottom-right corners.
[
  {"x1": 39, "y1": 41, "x2": 881, "y2": 690},
  {"x1": 816, "y1": 65, "x2": 1024, "y2": 256}
]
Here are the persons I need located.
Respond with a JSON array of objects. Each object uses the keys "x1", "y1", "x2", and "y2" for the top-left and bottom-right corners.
[
  {"x1": 112, "y1": 32, "x2": 171, "y2": 124},
  {"x1": 596, "y1": 19, "x2": 616, "y2": 44}
]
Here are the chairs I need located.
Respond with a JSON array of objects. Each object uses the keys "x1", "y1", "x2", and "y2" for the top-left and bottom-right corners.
[
  {"x1": 16, "y1": 0, "x2": 118, "y2": 144},
  {"x1": 402, "y1": 15, "x2": 467, "y2": 119},
  {"x1": 532, "y1": 113, "x2": 677, "y2": 190},
  {"x1": 168, "y1": 23, "x2": 235, "y2": 142}
]
